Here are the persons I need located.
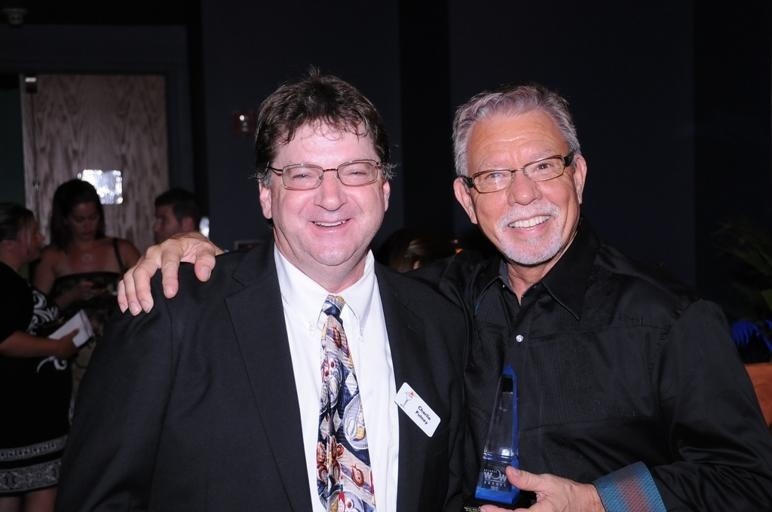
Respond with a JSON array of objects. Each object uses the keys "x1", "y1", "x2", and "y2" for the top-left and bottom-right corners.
[
  {"x1": 55, "y1": 75, "x2": 486, "y2": 511},
  {"x1": 114, "y1": 89, "x2": 772, "y2": 512},
  {"x1": 1, "y1": 179, "x2": 206, "y2": 512},
  {"x1": 374, "y1": 227, "x2": 772, "y2": 370}
]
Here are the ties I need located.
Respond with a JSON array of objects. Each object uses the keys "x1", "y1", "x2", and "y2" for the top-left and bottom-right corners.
[{"x1": 317, "y1": 295, "x2": 375, "y2": 511}]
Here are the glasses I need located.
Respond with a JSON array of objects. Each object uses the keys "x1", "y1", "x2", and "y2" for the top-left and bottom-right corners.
[
  {"x1": 461, "y1": 150, "x2": 574, "y2": 193},
  {"x1": 268, "y1": 160, "x2": 385, "y2": 192}
]
